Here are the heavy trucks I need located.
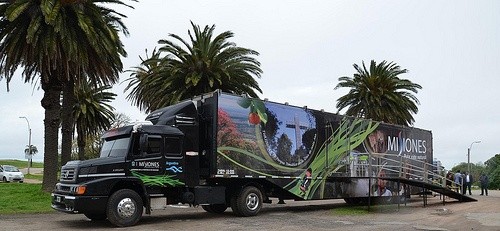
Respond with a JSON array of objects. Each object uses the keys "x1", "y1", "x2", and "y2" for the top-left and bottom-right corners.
[{"x1": 49, "y1": 91, "x2": 433, "y2": 229}]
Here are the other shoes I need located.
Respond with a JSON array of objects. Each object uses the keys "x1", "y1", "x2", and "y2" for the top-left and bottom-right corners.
[
  {"x1": 463, "y1": 193, "x2": 472, "y2": 195},
  {"x1": 479, "y1": 193, "x2": 488, "y2": 196}
]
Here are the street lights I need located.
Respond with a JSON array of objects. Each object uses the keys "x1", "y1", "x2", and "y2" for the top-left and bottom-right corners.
[
  {"x1": 466, "y1": 138, "x2": 482, "y2": 174},
  {"x1": 19, "y1": 116, "x2": 31, "y2": 176}
]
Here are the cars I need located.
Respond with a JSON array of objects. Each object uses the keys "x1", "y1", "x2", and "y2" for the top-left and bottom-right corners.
[{"x1": 0, "y1": 163, "x2": 24, "y2": 183}]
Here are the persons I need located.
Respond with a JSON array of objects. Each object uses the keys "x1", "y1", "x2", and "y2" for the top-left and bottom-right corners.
[
  {"x1": 478, "y1": 172, "x2": 488, "y2": 196},
  {"x1": 300, "y1": 168, "x2": 312, "y2": 196},
  {"x1": 446, "y1": 170, "x2": 473, "y2": 195},
  {"x1": 371, "y1": 169, "x2": 393, "y2": 196}
]
